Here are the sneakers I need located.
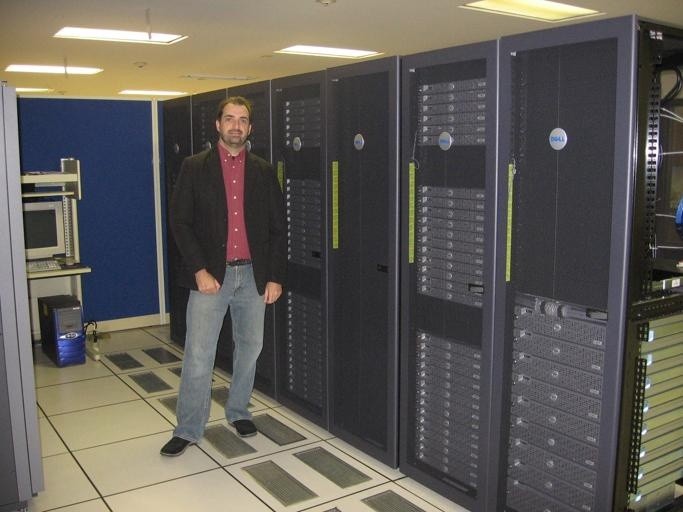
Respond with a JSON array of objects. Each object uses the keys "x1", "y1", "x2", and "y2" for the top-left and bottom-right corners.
[
  {"x1": 230, "y1": 419, "x2": 258, "y2": 437},
  {"x1": 160, "y1": 438, "x2": 195, "y2": 457}
]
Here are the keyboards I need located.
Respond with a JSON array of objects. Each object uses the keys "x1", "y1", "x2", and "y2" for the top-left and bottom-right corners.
[{"x1": 26, "y1": 259, "x2": 61, "y2": 272}]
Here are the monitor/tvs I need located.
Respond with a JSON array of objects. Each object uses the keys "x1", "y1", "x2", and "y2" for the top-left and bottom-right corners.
[{"x1": 22, "y1": 201, "x2": 66, "y2": 260}]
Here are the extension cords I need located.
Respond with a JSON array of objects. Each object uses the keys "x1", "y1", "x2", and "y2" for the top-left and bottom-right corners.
[{"x1": 85, "y1": 341, "x2": 101, "y2": 361}]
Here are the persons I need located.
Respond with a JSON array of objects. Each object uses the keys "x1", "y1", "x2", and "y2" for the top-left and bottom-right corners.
[{"x1": 158, "y1": 93, "x2": 289, "y2": 456}]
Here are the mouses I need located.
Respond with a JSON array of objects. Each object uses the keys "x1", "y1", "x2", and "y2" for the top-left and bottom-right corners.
[{"x1": 64, "y1": 257, "x2": 75, "y2": 266}]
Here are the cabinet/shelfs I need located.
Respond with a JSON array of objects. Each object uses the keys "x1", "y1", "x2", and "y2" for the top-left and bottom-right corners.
[{"x1": 158, "y1": 11, "x2": 682, "y2": 512}]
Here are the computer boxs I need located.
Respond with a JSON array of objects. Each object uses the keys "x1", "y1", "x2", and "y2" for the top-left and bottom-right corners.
[{"x1": 36, "y1": 294, "x2": 86, "y2": 368}]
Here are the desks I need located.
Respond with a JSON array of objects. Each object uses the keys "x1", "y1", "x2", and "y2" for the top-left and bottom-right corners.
[{"x1": 22, "y1": 159, "x2": 95, "y2": 345}]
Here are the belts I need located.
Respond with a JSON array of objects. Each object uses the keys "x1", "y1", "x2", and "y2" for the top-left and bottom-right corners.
[{"x1": 226, "y1": 259, "x2": 252, "y2": 268}]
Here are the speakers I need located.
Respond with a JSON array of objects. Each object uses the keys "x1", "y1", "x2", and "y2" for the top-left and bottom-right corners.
[{"x1": 61, "y1": 156, "x2": 81, "y2": 201}]
